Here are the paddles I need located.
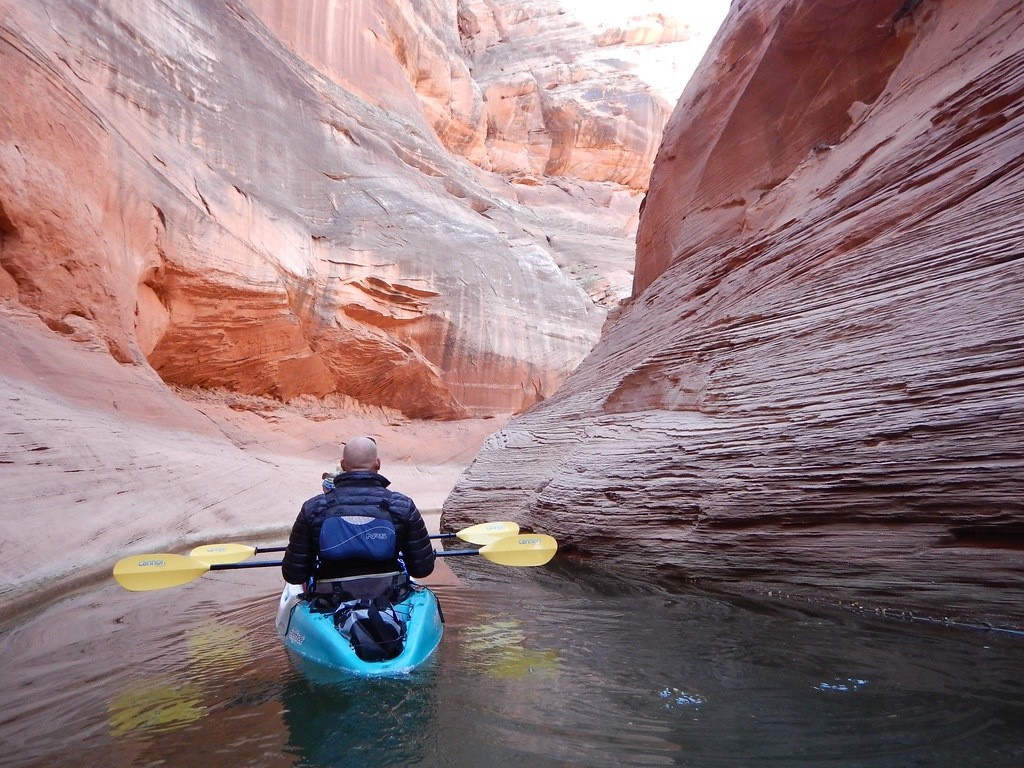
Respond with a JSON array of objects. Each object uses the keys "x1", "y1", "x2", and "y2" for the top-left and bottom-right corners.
[
  {"x1": 111, "y1": 532, "x2": 559, "y2": 589},
  {"x1": 188, "y1": 520, "x2": 521, "y2": 566}
]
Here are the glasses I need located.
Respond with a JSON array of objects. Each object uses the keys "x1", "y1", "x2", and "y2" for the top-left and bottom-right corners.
[{"x1": 366, "y1": 436, "x2": 377, "y2": 444}]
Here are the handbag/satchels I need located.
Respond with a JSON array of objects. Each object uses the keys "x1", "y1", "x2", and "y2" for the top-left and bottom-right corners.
[{"x1": 333, "y1": 598, "x2": 407, "y2": 662}]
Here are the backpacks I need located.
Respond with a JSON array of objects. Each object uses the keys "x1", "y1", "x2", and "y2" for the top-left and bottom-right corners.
[{"x1": 317, "y1": 488, "x2": 404, "y2": 606}]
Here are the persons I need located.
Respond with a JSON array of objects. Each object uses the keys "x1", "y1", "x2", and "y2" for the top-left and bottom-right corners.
[
  {"x1": 282, "y1": 437, "x2": 434, "y2": 609},
  {"x1": 321, "y1": 464, "x2": 346, "y2": 494}
]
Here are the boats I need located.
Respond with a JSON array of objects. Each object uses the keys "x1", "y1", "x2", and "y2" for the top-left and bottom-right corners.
[{"x1": 275, "y1": 581, "x2": 444, "y2": 677}]
